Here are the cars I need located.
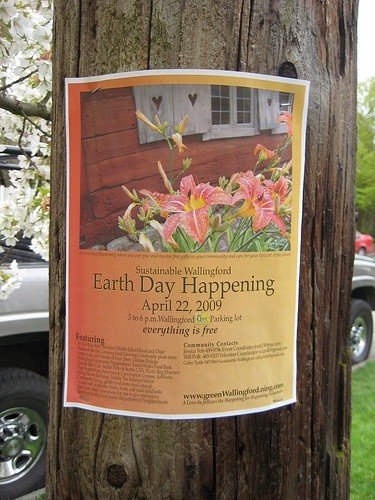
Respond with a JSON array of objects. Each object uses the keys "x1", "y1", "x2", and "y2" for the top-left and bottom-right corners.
[{"x1": 354, "y1": 229, "x2": 375, "y2": 256}]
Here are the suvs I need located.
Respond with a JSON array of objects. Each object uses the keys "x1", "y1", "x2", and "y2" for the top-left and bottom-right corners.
[{"x1": 0, "y1": 146, "x2": 375, "y2": 500}]
